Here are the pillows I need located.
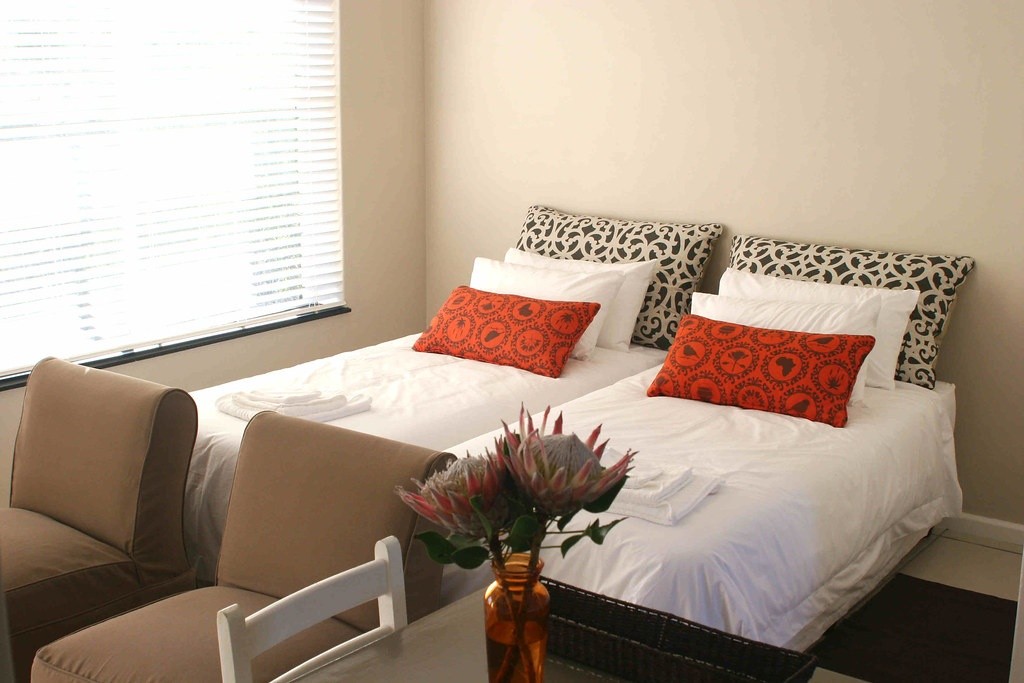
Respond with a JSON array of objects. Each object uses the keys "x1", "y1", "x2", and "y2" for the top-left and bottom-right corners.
[
  {"x1": 646, "y1": 313, "x2": 876, "y2": 428},
  {"x1": 728, "y1": 235, "x2": 975, "y2": 390},
  {"x1": 505, "y1": 247, "x2": 657, "y2": 351},
  {"x1": 412, "y1": 286, "x2": 602, "y2": 378},
  {"x1": 470, "y1": 258, "x2": 625, "y2": 362},
  {"x1": 691, "y1": 291, "x2": 882, "y2": 408},
  {"x1": 717, "y1": 268, "x2": 921, "y2": 390},
  {"x1": 517, "y1": 205, "x2": 724, "y2": 352}
]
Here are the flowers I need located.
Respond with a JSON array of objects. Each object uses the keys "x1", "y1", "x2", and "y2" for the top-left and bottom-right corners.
[{"x1": 393, "y1": 404, "x2": 640, "y2": 683}]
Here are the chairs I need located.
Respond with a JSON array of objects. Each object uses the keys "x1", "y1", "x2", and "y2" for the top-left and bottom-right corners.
[
  {"x1": 31, "y1": 412, "x2": 460, "y2": 683},
  {"x1": 0, "y1": 356, "x2": 200, "y2": 683},
  {"x1": 217, "y1": 536, "x2": 410, "y2": 683}
]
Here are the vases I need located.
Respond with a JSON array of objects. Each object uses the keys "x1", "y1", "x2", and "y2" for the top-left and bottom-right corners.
[{"x1": 484, "y1": 554, "x2": 549, "y2": 683}]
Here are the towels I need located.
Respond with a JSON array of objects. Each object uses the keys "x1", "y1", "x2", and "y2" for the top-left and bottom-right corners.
[
  {"x1": 213, "y1": 385, "x2": 374, "y2": 423},
  {"x1": 599, "y1": 448, "x2": 723, "y2": 529}
]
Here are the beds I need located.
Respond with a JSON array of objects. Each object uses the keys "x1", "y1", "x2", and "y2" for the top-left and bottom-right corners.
[
  {"x1": 186, "y1": 332, "x2": 669, "y2": 608},
  {"x1": 437, "y1": 362, "x2": 965, "y2": 654}
]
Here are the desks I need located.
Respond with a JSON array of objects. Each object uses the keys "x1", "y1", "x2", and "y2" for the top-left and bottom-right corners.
[{"x1": 290, "y1": 583, "x2": 871, "y2": 683}]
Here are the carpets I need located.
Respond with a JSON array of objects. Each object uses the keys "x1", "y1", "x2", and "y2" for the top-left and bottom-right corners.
[{"x1": 811, "y1": 572, "x2": 1018, "y2": 683}]
